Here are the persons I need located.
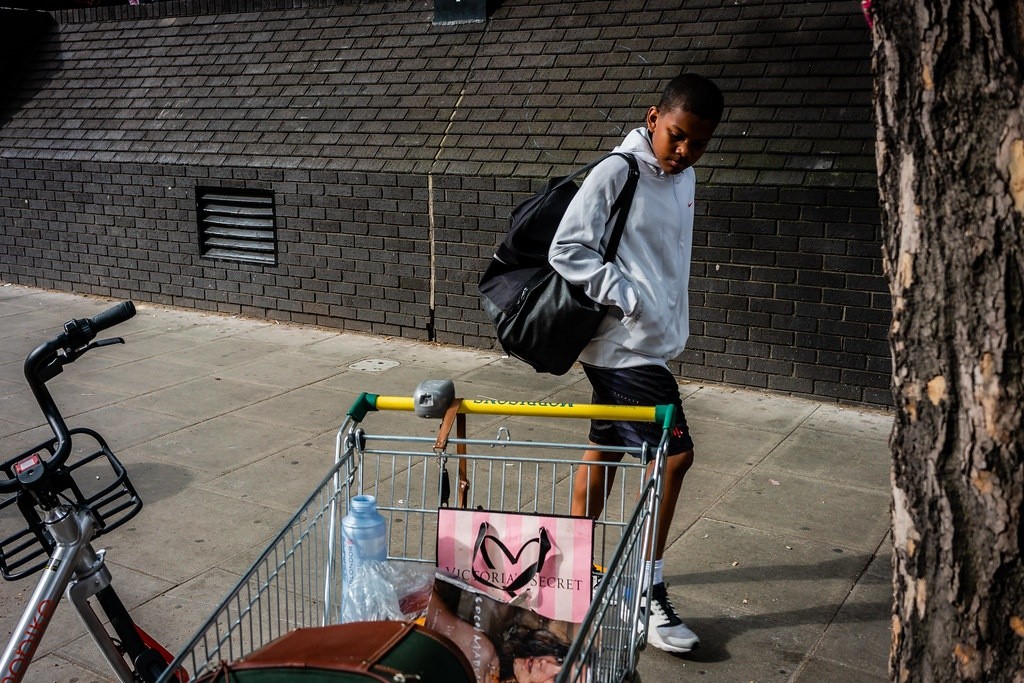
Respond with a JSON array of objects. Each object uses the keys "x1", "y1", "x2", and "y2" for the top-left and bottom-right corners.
[
  {"x1": 426, "y1": 592, "x2": 596, "y2": 683},
  {"x1": 548, "y1": 73, "x2": 724, "y2": 652}
]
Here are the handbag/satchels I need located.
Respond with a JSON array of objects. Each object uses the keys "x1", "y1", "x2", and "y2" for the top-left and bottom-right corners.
[
  {"x1": 192, "y1": 620, "x2": 477, "y2": 683},
  {"x1": 476, "y1": 152, "x2": 640, "y2": 376},
  {"x1": 437, "y1": 507, "x2": 593, "y2": 623}
]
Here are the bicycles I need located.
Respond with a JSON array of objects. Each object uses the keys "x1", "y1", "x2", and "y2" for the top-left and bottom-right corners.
[{"x1": 0, "y1": 299, "x2": 193, "y2": 683}]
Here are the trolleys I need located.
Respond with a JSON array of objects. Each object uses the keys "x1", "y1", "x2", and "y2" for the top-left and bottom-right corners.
[{"x1": 146, "y1": 381, "x2": 680, "y2": 683}]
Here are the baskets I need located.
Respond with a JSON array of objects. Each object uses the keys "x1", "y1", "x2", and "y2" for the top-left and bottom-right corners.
[{"x1": 0, "y1": 429, "x2": 143, "y2": 582}]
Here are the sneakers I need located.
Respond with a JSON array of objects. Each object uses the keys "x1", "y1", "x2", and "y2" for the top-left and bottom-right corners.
[
  {"x1": 620, "y1": 581, "x2": 702, "y2": 654},
  {"x1": 591, "y1": 570, "x2": 626, "y2": 605}
]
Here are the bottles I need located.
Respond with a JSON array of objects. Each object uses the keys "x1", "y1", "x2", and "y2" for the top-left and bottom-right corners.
[{"x1": 340, "y1": 495, "x2": 387, "y2": 626}]
